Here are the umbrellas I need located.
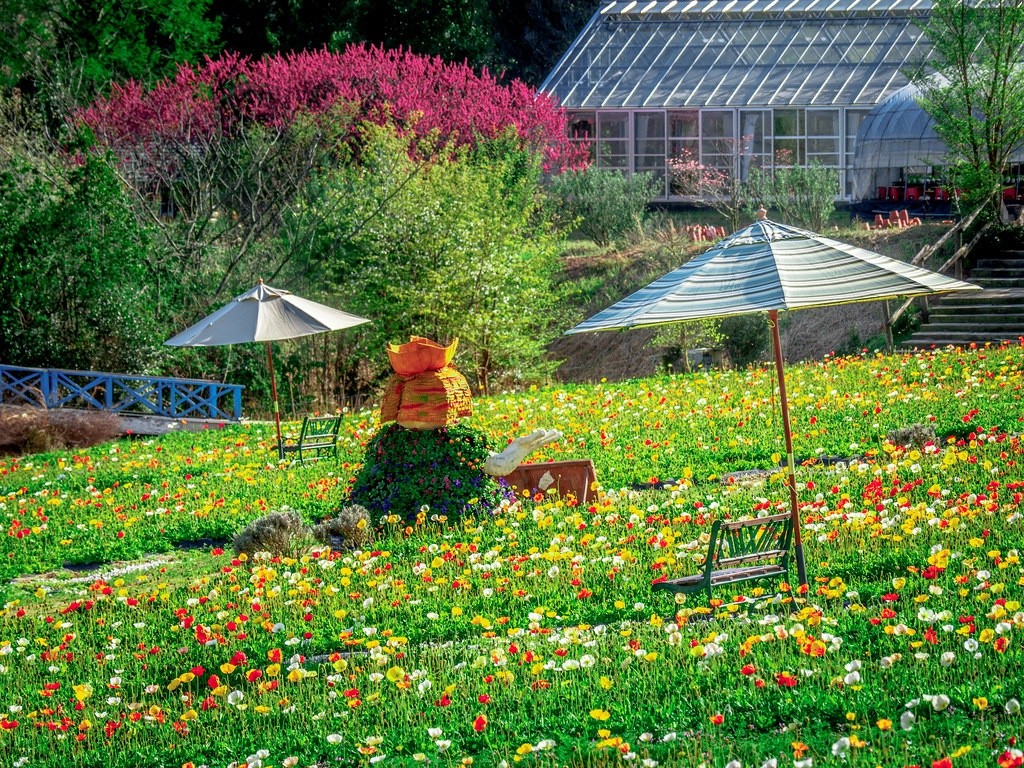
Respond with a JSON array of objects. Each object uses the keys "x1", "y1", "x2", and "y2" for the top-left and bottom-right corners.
[
  {"x1": 163, "y1": 276, "x2": 372, "y2": 463},
  {"x1": 563, "y1": 201, "x2": 985, "y2": 604}
]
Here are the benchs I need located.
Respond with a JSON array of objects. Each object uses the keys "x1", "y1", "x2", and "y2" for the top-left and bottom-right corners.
[
  {"x1": 271, "y1": 412, "x2": 343, "y2": 466},
  {"x1": 651, "y1": 511, "x2": 803, "y2": 622}
]
